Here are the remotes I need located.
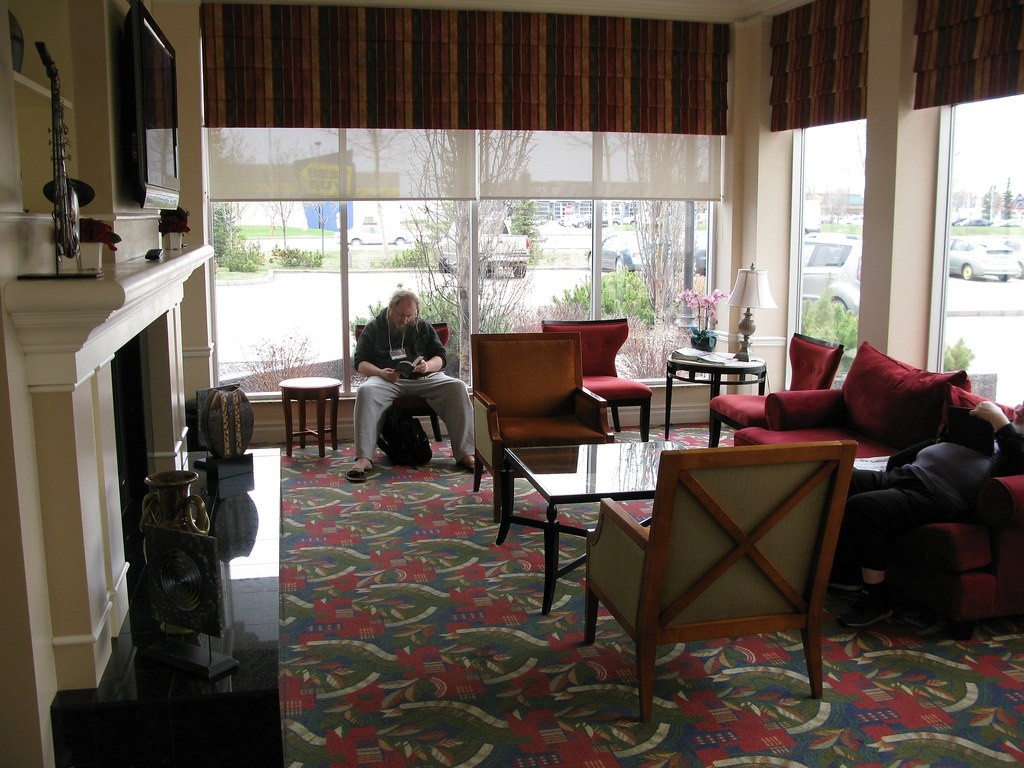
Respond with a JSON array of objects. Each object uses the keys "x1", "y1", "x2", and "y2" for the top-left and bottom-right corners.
[{"x1": 145, "y1": 248, "x2": 163, "y2": 260}]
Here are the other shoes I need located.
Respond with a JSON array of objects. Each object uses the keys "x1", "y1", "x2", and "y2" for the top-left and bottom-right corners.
[
  {"x1": 825, "y1": 571, "x2": 866, "y2": 591},
  {"x1": 837, "y1": 594, "x2": 893, "y2": 630}
]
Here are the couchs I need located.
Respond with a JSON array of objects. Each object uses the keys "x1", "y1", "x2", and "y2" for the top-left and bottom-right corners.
[{"x1": 734, "y1": 386, "x2": 1024, "y2": 638}]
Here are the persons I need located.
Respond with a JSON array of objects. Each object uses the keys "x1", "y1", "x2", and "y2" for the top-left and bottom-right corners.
[
  {"x1": 345, "y1": 291, "x2": 487, "y2": 483},
  {"x1": 830, "y1": 401, "x2": 1024, "y2": 627}
]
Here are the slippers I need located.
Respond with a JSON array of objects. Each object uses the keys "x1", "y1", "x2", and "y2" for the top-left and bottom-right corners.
[
  {"x1": 346, "y1": 467, "x2": 367, "y2": 483},
  {"x1": 458, "y1": 458, "x2": 488, "y2": 474}
]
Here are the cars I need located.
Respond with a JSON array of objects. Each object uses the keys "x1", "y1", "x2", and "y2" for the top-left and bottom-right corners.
[
  {"x1": 335, "y1": 220, "x2": 418, "y2": 246},
  {"x1": 952, "y1": 217, "x2": 994, "y2": 226},
  {"x1": 1002, "y1": 238, "x2": 1024, "y2": 280},
  {"x1": 588, "y1": 233, "x2": 683, "y2": 274},
  {"x1": 557, "y1": 212, "x2": 708, "y2": 229},
  {"x1": 948, "y1": 236, "x2": 1020, "y2": 282}
]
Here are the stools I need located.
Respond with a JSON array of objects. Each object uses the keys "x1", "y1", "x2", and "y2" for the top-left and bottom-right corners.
[{"x1": 278, "y1": 377, "x2": 342, "y2": 459}]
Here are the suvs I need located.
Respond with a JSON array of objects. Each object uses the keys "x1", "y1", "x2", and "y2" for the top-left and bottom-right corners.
[{"x1": 802, "y1": 230, "x2": 862, "y2": 317}]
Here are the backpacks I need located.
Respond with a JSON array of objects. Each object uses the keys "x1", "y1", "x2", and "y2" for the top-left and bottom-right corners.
[{"x1": 376, "y1": 407, "x2": 432, "y2": 468}]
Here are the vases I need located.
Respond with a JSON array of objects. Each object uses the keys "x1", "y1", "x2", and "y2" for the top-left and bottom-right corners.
[
  {"x1": 691, "y1": 337, "x2": 717, "y2": 353},
  {"x1": 136, "y1": 470, "x2": 211, "y2": 636},
  {"x1": 80, "y1": 242, "x2": 104, "y2": 271},
  {"x1": 165, "y1": 233, "x2": 182, "y2": 250}
]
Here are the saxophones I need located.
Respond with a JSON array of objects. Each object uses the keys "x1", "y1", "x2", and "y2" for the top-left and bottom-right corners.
[{"x1": 32, "y1": 36, "x2": 97, "y2": 260}]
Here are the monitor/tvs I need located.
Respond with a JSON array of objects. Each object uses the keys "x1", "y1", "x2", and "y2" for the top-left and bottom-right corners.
[{"x1": 123, "y1": 0, "x2": 181, "y2": 210}]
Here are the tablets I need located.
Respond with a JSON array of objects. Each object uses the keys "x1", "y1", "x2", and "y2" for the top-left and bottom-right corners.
[{"x1": 947, "y1": 406, "x2": 995, "y2": 456}]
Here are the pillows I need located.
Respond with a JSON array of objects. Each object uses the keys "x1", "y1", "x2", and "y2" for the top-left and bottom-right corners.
[
  {"x1": 937, "y1": 383, "x2": 1019, "y2": 441},
  {"x1": 839, "y1": 342, "x2": 968, "y2": 450}
]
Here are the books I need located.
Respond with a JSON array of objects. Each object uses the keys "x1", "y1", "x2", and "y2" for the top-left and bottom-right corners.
[
  {"x1": 671, "y1": 346, "x2": 738, "y2": 364},
  {"x1": 394, "y1": 356, "x2": 424, "y2": 378}
]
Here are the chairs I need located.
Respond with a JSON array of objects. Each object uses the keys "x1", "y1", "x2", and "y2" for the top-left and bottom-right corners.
[
  {"x1": 584, "y1": 441, "x2": 855, "y2": 722},
  {"x1": 356, "y1": 321, "x2": 448, "y2": 443},
  {"x1": 470, "y1": 331, "x2": 616, "y2": 522},
  {"x1": 707, "y1": 333, "x2": 844, "y2": 449},
  {"x1": 541, "y1": 319, "x2": 653, "y2": 441}
]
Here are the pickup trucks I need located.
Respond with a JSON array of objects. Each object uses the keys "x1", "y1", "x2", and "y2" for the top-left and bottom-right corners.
[{"x1": 437, "y1": 216, "x2": 530, "y2": 278}]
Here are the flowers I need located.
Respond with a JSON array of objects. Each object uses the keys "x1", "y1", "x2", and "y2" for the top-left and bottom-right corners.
[
  {"x1": 157, "y1": 207, "x2": 194, "y2": 234},
  {"x1": 675, "y1": 285, "x2": 727, "y2": 340},
  {"x1": 79, "y1": 217, "x2": 122, "y2": 252}
]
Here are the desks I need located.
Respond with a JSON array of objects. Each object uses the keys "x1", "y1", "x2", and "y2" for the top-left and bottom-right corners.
[
  {"x1": 664, "y1": 355, "x2": 767, "y2": 448},
  {"x1": 498, "y1": 440, "x2": 692, "y2": 613},
  {"x1": 51, "y1": 449, "x2": 282, "y2": 767}
]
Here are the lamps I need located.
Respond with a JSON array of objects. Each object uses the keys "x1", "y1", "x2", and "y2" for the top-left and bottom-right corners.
[{"x1": 729, "y1": 264, "x2": 777, "y2": 360}]
